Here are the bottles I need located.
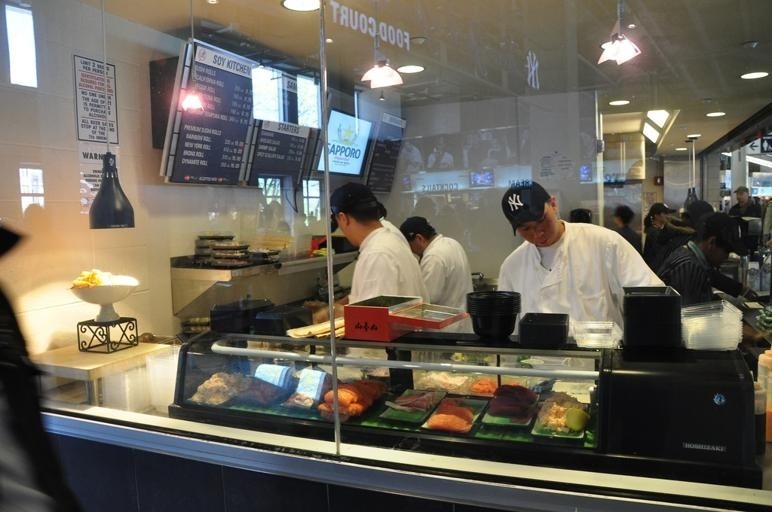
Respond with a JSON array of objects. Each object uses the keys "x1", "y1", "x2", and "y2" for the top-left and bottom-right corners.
[
  {"x1": 738, "y1": 252, "x2": 770, "y2": 293},
  {"x1": 754, "y1": 382, "x2": 766, "y2": 455},
  {"x1": 757, "y1": 350, "x2": 772, "y2": 443}
]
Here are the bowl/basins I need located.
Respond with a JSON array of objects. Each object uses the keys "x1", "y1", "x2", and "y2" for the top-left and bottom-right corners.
[{"x1": 466, "y1": 291, "x2": 519, "y2": 337}]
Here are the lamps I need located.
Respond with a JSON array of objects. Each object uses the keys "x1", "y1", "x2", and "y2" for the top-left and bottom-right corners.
[
  {"x1": 181, "y1": 0, "x2": 202, "y2": 112},
  {"x1": 361, "y1": 1, "x2": 403, "y2": 88},
  {"x1": 598, "y1": 0, "x2": 640, "y2": 66},
  {"x1": 689, "y1": 137, "x2": 699, "y2": 207},
  {"x1": 88, "y1": 1, "x2": 135, "y2": 229},
  {"x1": 684, "y1": 141, "x2": 692, "y2": 209}
]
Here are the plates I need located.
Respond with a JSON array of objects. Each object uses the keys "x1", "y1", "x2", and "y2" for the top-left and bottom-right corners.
[
  {"x1": 382, "y1": 389, "x2": 446, "y2": 424},
  {"x1": 520, "y1": 312, "x2": 570, "y2": 346},
  {"x1": 531, "y1": 398, "x2": 589, "y2": 439},
  {"x1": 420, "y1": 394, "x2": 488, "y2": 433},
  {"x1": 621, "y1": 284, "x2": 681, "y2": 358},
  {"x1": 481, "y1": 392, "x2": 540, "y2": 426}
]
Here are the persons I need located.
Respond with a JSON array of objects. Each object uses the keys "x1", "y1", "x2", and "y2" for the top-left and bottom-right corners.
[
  {"x1": 401, "y1": 141, "x2": 422, "y2": 171},
  {"x1": 330, "y1": 182, "x2": 427, "y2": 328},
  {"x1": 465, "y1": 128, "x2": 530, "y2": 169},
  {"x1": 612, "y1": 205, "x2": 642, "y2": 255},
  {"x1": 398, "y1": 216, "x2": 474, "y2": 334},
  {"x1": 427, "y1": 136, "x2": 454, "y2": 168},
  {"x1": 331, "y1": 203, "x2": 431, "y2": 306},
  {"x1": 497, "y1": 182, "x2": 666, "y2": 347},
  {"x1": 642, "y1": 187, "x2": 772, "y2": 306}
]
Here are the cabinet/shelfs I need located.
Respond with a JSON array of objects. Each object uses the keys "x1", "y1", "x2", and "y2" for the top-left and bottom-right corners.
[
  {"x1": 604, "y1": 132, "x2": 646, "y2": 183},
  {"x1": 170, "y1": 329, "x2": 758, "y2": 488}
]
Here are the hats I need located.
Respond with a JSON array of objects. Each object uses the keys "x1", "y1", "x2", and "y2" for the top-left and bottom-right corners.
[
  {"x1": 733, "y1": 186, "x2": 749, "y2": 193},
  {"x1": 701, "y1": 212, "x2": 749, "y2": 257},
  {"x1": 649, "y1": 202, "x2": 677, "y2": 215},
  {"x1": 501, "y1": 180, "x2": 549, "y2": 236},
  {"x1": 329, "y1": 181, "x2": 377, "y2": 233},
  {"x1": 400, "y1": 216, "x2": 428, "y2": 237}
]
